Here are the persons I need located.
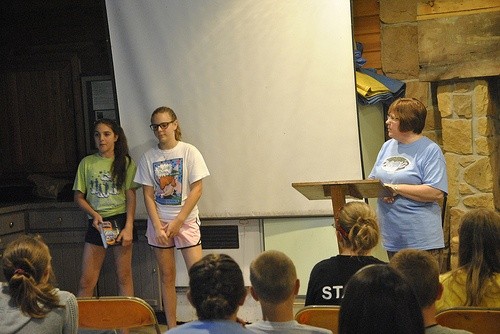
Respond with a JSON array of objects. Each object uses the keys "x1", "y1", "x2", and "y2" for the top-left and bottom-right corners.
[
  {"x1": 0, "y1": 233, "x2": 79, "y2": 334},
  {"x1": 244, "y1": 249, "x2": 334, "y2": 334},
  {"x1": 132, "y1": 106, "x2": 211, "y2": 330},
  {"x1": 162, "y1": 253, "x2": 253, "y2": 334},
  {"x1": 434, "y1": 208, "x2": 500, "y2": 312},
  {"x1": 386, "y1": 248, "x2": 473, "y2": 334},
  {"x1": 73, "y1": 118, "x2": 143, "y2": 334},
  {"x1": 305, "y1": 201, "x2": 388, "y2": 306},
  {"x1": 337, "y1": 263, "x2": 426, "y2": 334},
  {"x1": 367, "y1": 97, "x2": 449, "y2": 273}
]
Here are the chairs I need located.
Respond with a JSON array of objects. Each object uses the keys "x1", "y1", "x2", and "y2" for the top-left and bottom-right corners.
[
  {"x1": 76, "y1": 296, "x2": 160, "y2": 334},
  {"x1": 433, "y1": 306, "x2": 500, "y2": 334},
  {"x1": 295, "y1": 305, "x2": 341, "y2": 334}
]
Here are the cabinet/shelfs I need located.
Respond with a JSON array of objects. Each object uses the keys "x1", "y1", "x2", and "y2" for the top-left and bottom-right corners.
[
  {"x1": 26, "y1": 202, "x2": 97, "y2": 297},
  {"x1": 0, "y1": 203, "x2": 26, "y2": 282},
  {"x1": 0, "y1": 51, "x2": 90, "y2": 191},
  {"x1": 97, "y1": 220, "x2": 163, "y2": 323}
]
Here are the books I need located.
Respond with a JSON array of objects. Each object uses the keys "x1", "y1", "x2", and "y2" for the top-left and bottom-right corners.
[{"x1": 98, "y1": 218, "x2": 123, "y2": 250}]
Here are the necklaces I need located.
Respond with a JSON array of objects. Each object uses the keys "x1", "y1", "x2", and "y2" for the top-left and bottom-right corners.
[{"x1": 159, "y1": 149, "x2": 171, "y2": 161}]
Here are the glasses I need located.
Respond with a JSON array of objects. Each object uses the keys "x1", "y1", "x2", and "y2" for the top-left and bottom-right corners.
[
  {"x1": 386, "y1": 113, "x2": 400, "y2": 121},
  {"x1": 149, "y1": 120, "x2": 173, "y2": 131}
]
